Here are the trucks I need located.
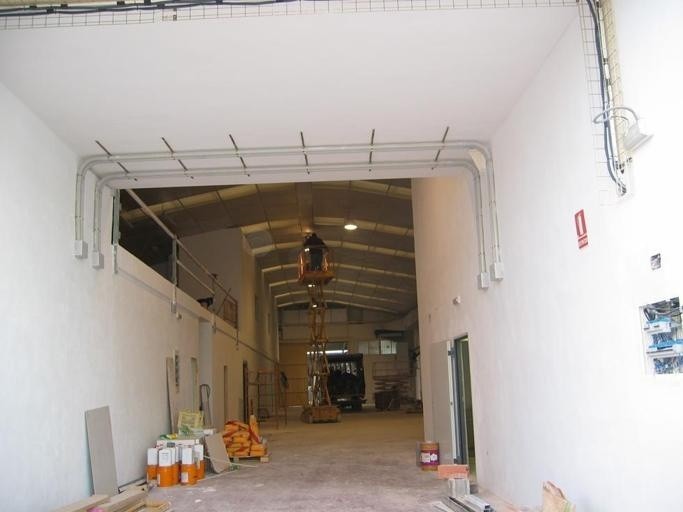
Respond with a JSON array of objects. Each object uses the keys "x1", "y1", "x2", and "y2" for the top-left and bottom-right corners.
[{"x1": 305, "y1": 353, "x2": 366, "y2": 411}]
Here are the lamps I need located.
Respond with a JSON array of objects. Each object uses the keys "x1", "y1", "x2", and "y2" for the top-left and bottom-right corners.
[{"x1": 344, "y1": 208, "x2": 357, "y2": 231}]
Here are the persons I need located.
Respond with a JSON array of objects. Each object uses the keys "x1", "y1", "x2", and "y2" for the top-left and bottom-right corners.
[{"x1": 304, "y1": 232, "x2": 328, "y2": 272}]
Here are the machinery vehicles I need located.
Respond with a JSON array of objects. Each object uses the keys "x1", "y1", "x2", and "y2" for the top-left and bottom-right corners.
[{"x1": 298, "y1": 244, "x2": 341, "y2": 424}]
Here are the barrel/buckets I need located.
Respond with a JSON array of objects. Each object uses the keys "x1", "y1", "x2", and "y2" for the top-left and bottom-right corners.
[{"x1": 418, "y1": 441, "x2": 441, "y2": 473}]
